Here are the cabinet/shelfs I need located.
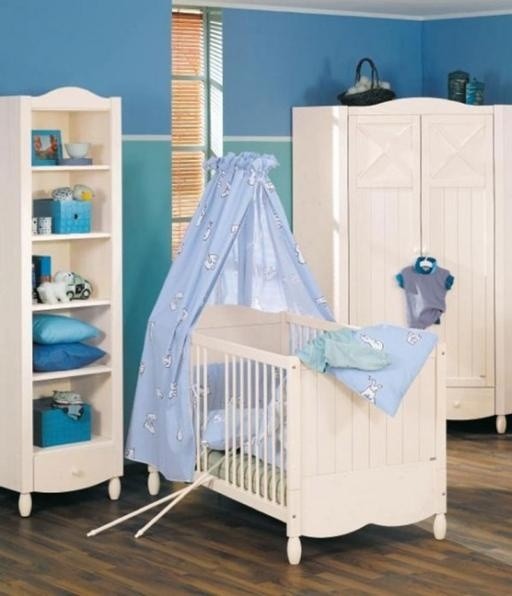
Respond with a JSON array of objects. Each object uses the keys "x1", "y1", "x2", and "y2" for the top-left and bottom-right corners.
[
  {"x1": 0, "y1": 86, "x2": 122, "y2": 517},
  {"x1": 292, "y1": 98, "x2": 512, "y2": 433}
]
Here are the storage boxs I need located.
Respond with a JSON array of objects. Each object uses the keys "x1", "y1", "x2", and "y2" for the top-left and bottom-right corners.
[
  {"x1": 49, "y1": 199, "x2": 91, "y2": 233},
  {"x1": 34, "y1": 398, "x2": 91, "y2": 448}
]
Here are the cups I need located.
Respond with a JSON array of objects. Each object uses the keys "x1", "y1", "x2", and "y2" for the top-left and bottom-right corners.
[
  {"x1": 32, "y1": 218, "x2": 37, "y2": 235},
  {"x1": 39, "y1": 217, "x2": 52, "y2": 235}
]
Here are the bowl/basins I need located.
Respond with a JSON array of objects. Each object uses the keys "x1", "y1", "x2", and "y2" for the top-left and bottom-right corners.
[{"x1": 64, "y1": 144, "x2": 90, "y2": 158}]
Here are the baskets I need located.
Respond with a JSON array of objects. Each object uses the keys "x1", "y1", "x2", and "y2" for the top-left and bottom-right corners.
[{"x1": 338, "y1": 58, "x2": 396, "y2": 106}]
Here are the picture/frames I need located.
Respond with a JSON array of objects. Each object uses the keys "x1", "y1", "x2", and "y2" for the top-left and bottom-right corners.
[{"x1": 32, "y1": 129, "x2": 63, "y2": 165}]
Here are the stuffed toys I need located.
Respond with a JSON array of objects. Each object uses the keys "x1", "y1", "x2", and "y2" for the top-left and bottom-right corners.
[{"x1": 37, "y1": 269, "x2": 74, "y2": 305}]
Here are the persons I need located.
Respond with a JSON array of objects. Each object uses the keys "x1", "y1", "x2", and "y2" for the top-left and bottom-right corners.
[
  {"x1": 46, "y1": 135, "x2": 57, "y2": 160},
  {"x1": 33, "y1": 136, "x2": 46, "y2": 160}
]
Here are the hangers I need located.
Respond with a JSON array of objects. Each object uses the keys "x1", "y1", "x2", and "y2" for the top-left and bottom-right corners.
[{"x1": 416, "y1": 251, "x2": 433, "y2": 271}]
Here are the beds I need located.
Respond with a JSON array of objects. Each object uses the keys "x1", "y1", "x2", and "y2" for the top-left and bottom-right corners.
[{"x1": 148, "y1": 304, "x2": 448, "y2": 566}]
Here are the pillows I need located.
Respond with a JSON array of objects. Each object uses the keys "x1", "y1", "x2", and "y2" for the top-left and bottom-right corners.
[
  {"x1": 203, "y1": 408, "x2": 264, "y2": 450},
  {"x1": 243, "y1": 433, "x2": 286, "y2": 467},
  {"x1": 34, "y1": 342, "x2": 106, "y2": 373},
  {"x1": 34, "y1": 314, "x2": 100, "y2": 345}
]
